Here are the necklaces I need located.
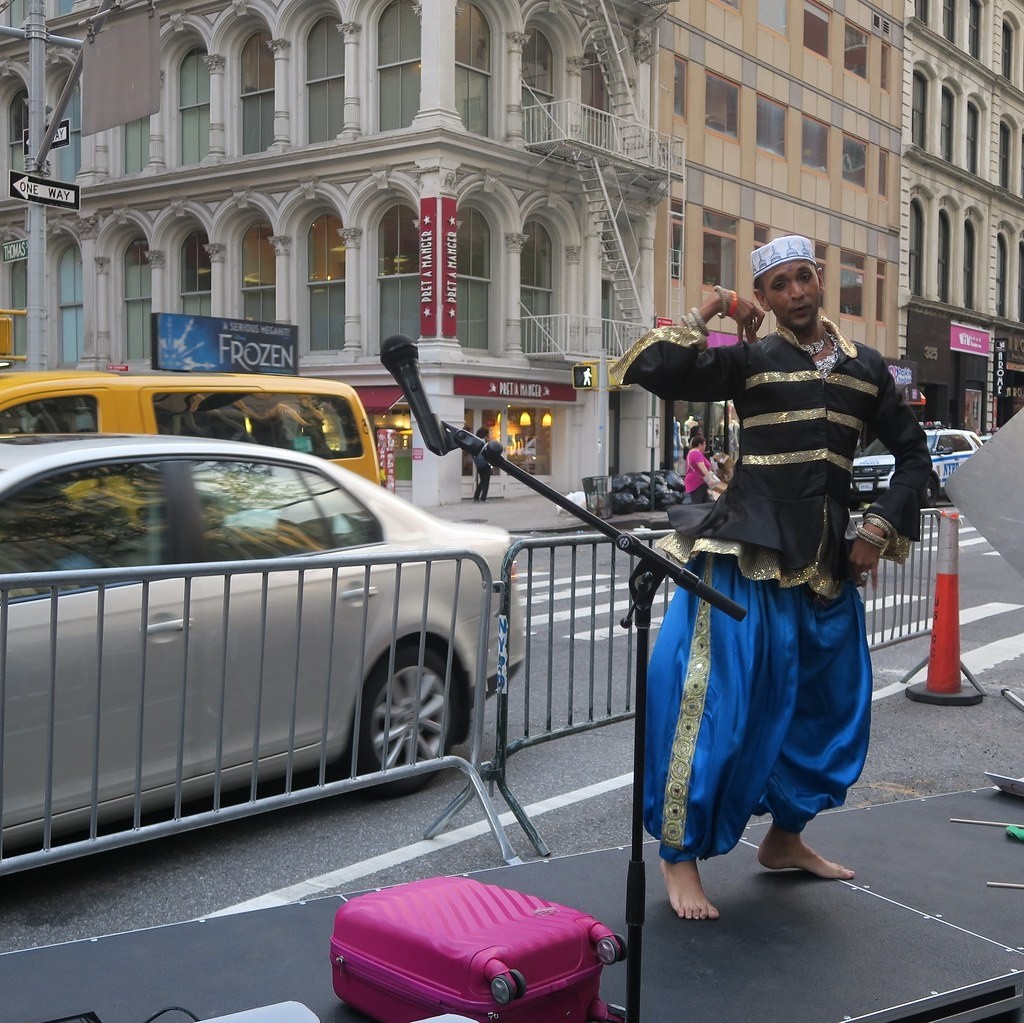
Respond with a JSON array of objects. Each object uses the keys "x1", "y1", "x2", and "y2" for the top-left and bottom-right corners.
[{"x1": 799, "y1": 330, "x2": 829, "y2": 356}]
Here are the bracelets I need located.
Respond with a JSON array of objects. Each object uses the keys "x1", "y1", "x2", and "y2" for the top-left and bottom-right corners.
[
  {"x1": 853, "y1": 516, "x2": 892, "y2": 549},
  {"x1": 681, "y1": 286, "x2": 737, "y2": 338}
]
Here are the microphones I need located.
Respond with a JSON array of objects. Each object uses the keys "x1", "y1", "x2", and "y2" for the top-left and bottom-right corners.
[{"x1": 381, "y1": 334, "x2": 445, "y2": 456}]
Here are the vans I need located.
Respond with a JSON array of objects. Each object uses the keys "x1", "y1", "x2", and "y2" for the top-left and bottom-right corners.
[{"x1": 1, "y1": 372, "x2": 381, "y2": 488}]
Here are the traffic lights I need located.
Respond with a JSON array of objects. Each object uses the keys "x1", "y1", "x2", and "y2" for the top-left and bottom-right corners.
[{"x1": 572, "y1": 365, "x2": 597, "y2": 389}]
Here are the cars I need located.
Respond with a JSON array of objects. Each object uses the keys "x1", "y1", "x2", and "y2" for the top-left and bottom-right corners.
[{"x1": 0, "y1": 432, "x2": 529, "y2": 853}]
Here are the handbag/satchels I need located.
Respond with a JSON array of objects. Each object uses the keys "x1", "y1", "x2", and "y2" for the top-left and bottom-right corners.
[{"x1": 703, "y1": 471, "x2": 720, "y2": 489}]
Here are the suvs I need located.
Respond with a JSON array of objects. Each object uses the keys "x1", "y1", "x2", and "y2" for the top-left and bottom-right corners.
[{"x1": 848, "y1": 422, "x2": 984, "y2": 512}]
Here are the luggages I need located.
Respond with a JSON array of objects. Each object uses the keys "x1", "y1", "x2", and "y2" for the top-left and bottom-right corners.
[{"x1": 330, "y1": 878, "x2": 625, "y2": 1023}]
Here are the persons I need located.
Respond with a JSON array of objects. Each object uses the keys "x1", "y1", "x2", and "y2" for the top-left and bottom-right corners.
[
  {"x1": 683, "y1": 416, "x2": 699, "y2": 460},
  {"x1": 688, "y1": 426, "x2": 711, "y2": 455},
  {"x1": 684, "y1": 435, "x2": 715, "y2": 504},
  {"x1": 473, "y1": 428, "x2": 491, "y2": 504},
  {"x1": 619, "y1": 234, "x2": 931, "y2": 922},
  {"x1": 673, "y1": 416, "x2": 682, "y2": 474}
]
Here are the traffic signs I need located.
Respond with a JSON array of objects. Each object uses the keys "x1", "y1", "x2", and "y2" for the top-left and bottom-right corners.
[
  {"x1": 22, "y1": 118, "x2": 69, "y2": 156},
  {"x1": 8, "y1": 169, "x2": 81, "y2": 212}
]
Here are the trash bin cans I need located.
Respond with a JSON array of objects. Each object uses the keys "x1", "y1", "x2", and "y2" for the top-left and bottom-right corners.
[{"x1": 582, "y1": 476, "x2": 613, "y2": 519}]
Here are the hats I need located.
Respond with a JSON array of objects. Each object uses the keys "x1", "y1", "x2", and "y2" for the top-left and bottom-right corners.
[{"x1": 752, "y1": 236, "x2": 816, "y2": 278}]
